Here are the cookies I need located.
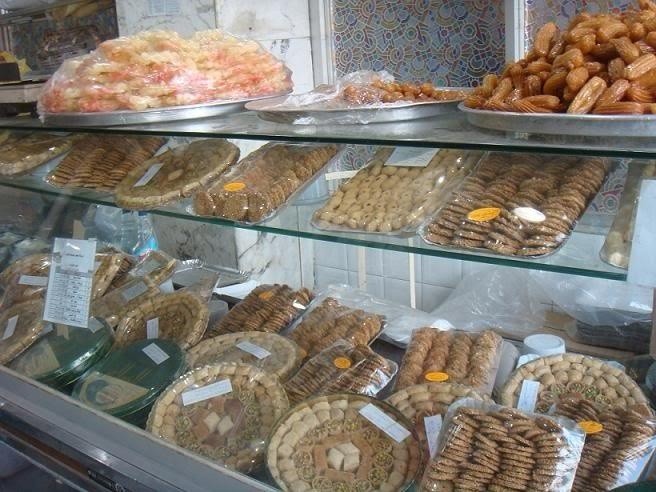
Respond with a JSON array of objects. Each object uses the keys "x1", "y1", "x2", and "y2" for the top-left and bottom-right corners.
[{"x1": 1, "y1": 131, "x2": 656, "y2": 492}]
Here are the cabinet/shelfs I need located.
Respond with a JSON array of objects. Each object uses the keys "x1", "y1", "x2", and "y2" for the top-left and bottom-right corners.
[{"x1": 0, "y1": 110, "x2": 656, "y2": 492}]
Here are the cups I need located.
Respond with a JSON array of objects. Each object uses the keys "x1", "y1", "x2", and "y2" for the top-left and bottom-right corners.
[
  {"x1": 521, "y1": 332, "x2": 567, "y2": 360},
  {"x1": 204, "y1": 299, "x2": 229, "y2": 329}
]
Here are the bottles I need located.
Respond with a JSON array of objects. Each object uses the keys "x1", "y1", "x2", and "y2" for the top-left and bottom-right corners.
[{"x1": 115, "y1": 208, "x2": 158, "y2": 258}]
